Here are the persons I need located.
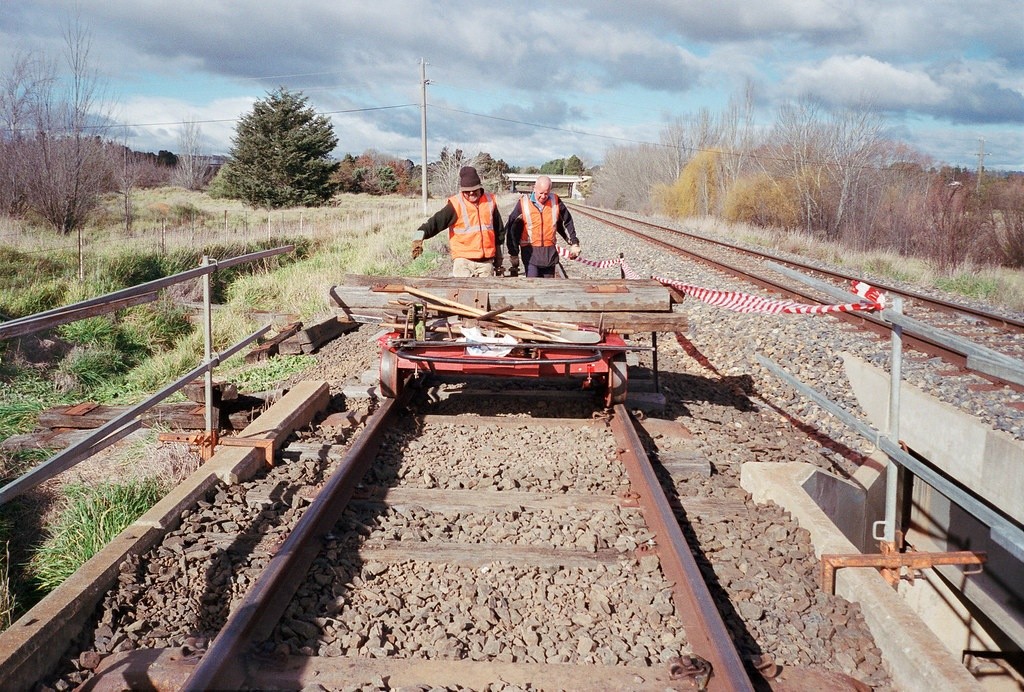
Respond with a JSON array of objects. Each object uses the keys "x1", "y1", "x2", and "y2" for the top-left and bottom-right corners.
[
  {"x1": 400, "y1": 163, "x2": 505, "y2": 278},
  {"x1": 505, "y1": 174, "x2": 582, "y2": 278}
]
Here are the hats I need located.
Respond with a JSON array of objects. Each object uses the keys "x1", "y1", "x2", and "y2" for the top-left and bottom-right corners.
[{"x1": 459, "y1": 166, "x2": 483, "y2": 191}]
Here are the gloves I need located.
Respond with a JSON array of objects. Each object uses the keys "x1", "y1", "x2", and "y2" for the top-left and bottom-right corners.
[
  {"x1": 412, "y1": 240, "x2": 423, "y2": 260},
  {"x1": 510, "y1": 255, "x2": 519, "y2": 267},
  {"x1": 492, "y1": 244, "x2": 504, "y2": 268},
  {"x1": 568, "y1": 244, "x2": 581, "y2": 259}
]
